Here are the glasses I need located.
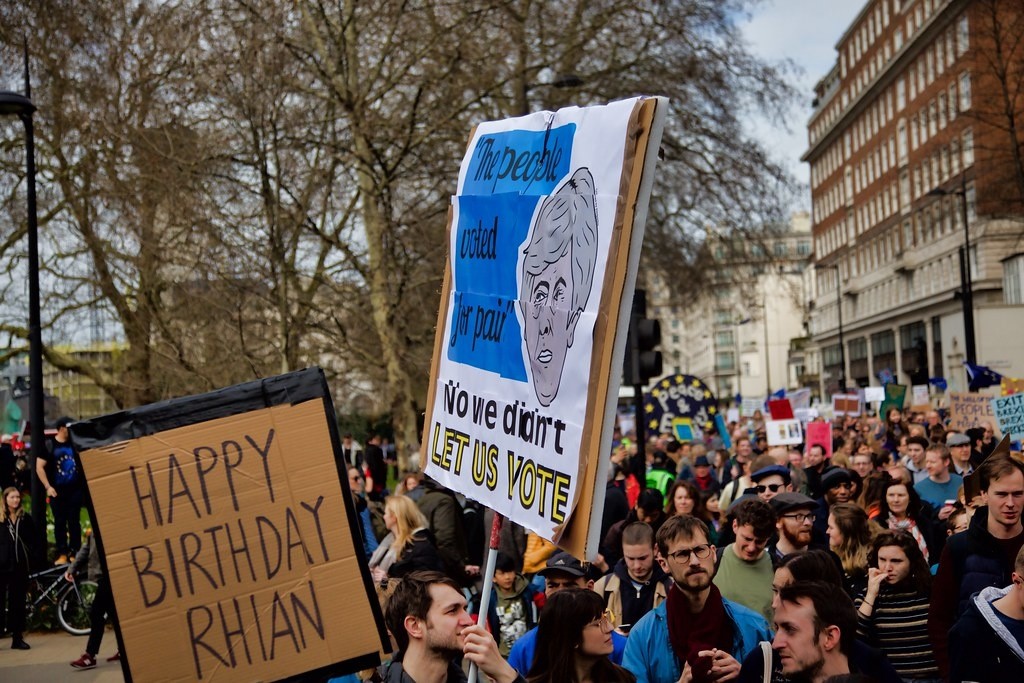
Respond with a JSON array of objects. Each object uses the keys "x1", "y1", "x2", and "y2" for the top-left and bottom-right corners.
[
  {"x1": 350, "y1": 475, "x2": 362, "y2": 482},
  {"x1": 666, "y1": 544, "x2": 712, "y2": 564},
  {"x1": 754, "y1": 483, "x2": 787, "y2": 493},
  {"x1": 780, "y1": 512, "x2": 816, "y2": 523}
]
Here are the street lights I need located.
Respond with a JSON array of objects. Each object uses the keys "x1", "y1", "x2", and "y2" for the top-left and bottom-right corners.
[
  {"x1": 814, "y1": 263, "x2": 846, "y2": 389},
  {"x1": 925, "y1": 187, "x2": 979, "y2": 392},
  {"x1": 0, "y1": 90, "x2": 46, "y2": 555}
]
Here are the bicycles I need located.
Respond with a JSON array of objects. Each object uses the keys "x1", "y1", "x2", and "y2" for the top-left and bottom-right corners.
[{"x1": 3, "y1": 548, "x2": 112, "y2": 636}]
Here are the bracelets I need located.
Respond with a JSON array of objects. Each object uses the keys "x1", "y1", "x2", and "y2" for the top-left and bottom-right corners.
[{"x1": 863, "y1": 599, "x2": 873, "y2": 606}]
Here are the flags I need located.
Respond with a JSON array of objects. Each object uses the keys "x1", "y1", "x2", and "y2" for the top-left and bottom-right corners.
[{"x1": 964, "y1": 362, "x2": 1005, "y2": 386}]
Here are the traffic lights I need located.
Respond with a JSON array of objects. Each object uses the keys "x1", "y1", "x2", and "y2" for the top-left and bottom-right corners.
[{"x1": 629, "y1": 290, "x2": 663, "y2": 386}]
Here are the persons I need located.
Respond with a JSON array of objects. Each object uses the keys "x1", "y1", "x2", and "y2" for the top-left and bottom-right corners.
[
  {"x1": 0, "y1": 433, "x2": 15, "y2": 492},
  {"x1": 0, "y1": 487, "x2": 35, "y2": 649},
  {"x1": 65, "y1": 529, "x2": 122, "y2": 668},
  {"x1": 790, "y1": 424, "x2": 799, "y2": 437},
  {"x1": 340, "y1": 405, "x2": 1024, "y2": 683},
  {"x1": 35, "y1": 416, "x2": 84, "y2": 565}
]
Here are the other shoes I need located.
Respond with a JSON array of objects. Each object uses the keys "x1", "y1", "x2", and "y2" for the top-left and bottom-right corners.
[
  {"x1": 54, "y1": 555, "x2": 67, "y2": 565},
  {"x1": 10, "y1": 640, "x2": 30, "y2": 650},
  {"x1": 69, "y1": 556, "x2": 76, "y2": 562}
]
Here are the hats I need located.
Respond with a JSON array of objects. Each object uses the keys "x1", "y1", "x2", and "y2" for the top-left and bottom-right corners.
[
  {"x1": 343, "y1": 432, "x2": 352, "y2": 439},
  {"x1": 536, "y1": 550, "x2": 588, "y2": 576},
  {"x1": 820, "y1": 465, "x2": 851, "y2": 491},
  {"x1": 692, "y1": 455, "x2": 711, "y2": 468},
  {"x1": 54, "y1": 415, "x2": 77, "y2": 430},
  {"x1": 750, "y1": 464, "x2": 791, "y2": 484},
  {"x1": 768, "y1": 491, "x2": 820, "y2": 519},
  {"x1": 947, "y1": 434, "x2": 971, "y2": 449}
]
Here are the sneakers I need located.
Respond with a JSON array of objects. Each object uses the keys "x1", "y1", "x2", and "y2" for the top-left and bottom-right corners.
[
  {"x1": 106, "y1": 651, "x2": 121, "y2": 663},
  {"x1": 70, "y1": 653, "x2": 97, "y2": 669}
]
